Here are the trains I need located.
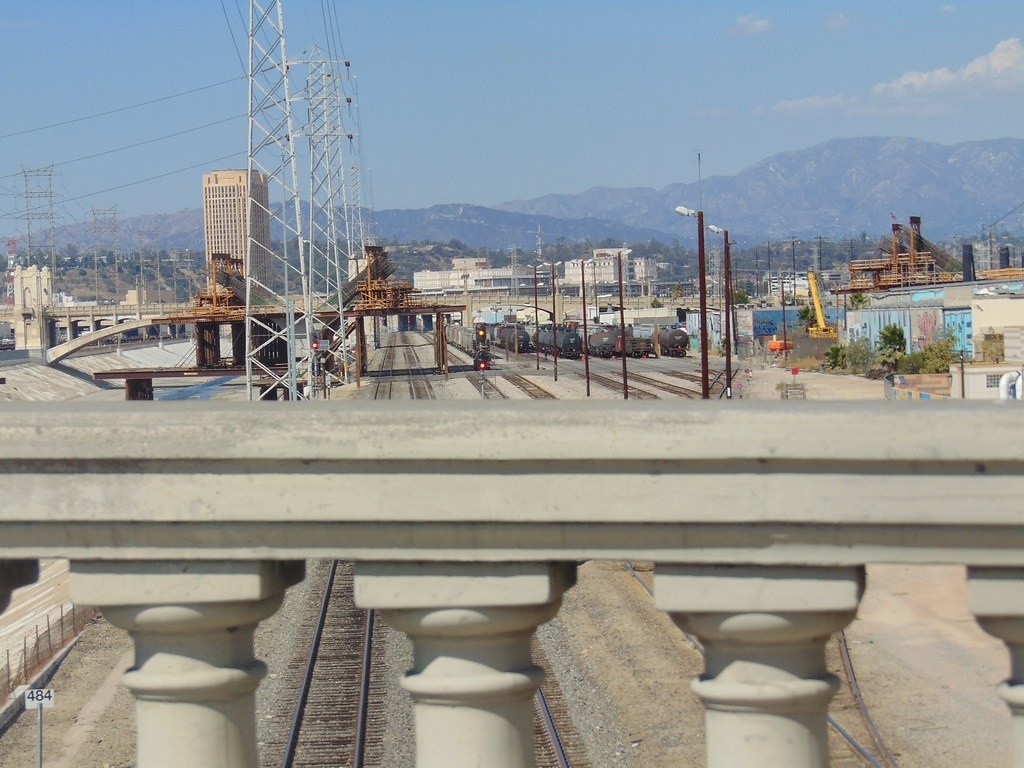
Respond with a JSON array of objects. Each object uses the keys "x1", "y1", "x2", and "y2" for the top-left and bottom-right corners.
[
  {"x1": 586, "y1": 324, "x2": 635, "y2": 360},
  {"x1": 476, "y1": 323, "x2": 530, "y2": 354},
  {"x1": 630, "y1": 324, "x2": 689, "y2": 358},
  {"x1": 532, "y1": 321, "x2": 582, "y2": 360}
]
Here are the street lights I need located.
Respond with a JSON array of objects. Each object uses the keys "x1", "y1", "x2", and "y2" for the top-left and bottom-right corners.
[
  {"x1": 617, "y1": 248, "x2": 632, "y2": 399},
  {"x1": 674, "y1": 205, "x2": 710, "y2": 399},
  {"x1": 811, "y1": 235, "x2": 832, "y2": 270},
  {"x1": 786, "y1": 235, "x2": 801, "y2": 301},
  {"x1": 762, "y1": 240, "x2": 777, "y2": 296},
  {"x1": 526, "y1": 264, "x2": 543, "y2": 372},
  {"x1": 570, "y1": 258, "x2": 593, "y2": 397},
  {"x1": 543, "y1": 261, "x2": 563, "y2": 382},
  {"x1": 707, "y1": 223, "x2": 733, "y2": 399}
]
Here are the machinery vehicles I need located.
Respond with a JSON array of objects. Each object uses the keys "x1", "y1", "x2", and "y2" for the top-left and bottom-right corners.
[{"x1": 806, "y1": 263, "x2": 838, "y2": 340}]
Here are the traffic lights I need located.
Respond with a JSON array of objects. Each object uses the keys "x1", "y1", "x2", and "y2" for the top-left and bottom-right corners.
[
  {"x1": 476, "y1": 323, "x2": 485, "y2": 341},
  {"x1": 478, "y1": 361, "x2": 487, "y2": 370},
  {"x1": 312, "y1": 333, "x2": 318, "y2": 348}
]
